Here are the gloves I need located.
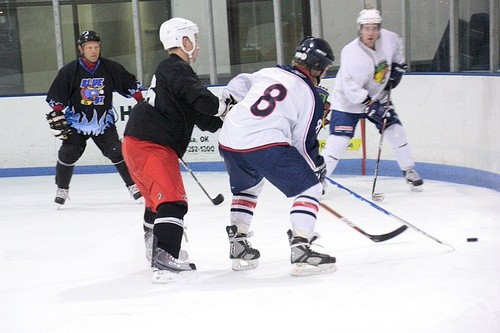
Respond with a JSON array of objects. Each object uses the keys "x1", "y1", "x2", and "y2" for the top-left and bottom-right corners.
[
  {"x1": 385, "y1": 63, "x2": 407, "y2": 90},
  {"x1": 365, "y1": 99, "x2": 394, "y2": 124},
  {"x1": 315, "y1": 156, "x2": 328, "y2": 182},
  {"x1": 196, "y1": 114, "x2": 223, "y2": 133},
  {"x1": 45, "y1": 109, "x2": 72, "y2": 140},
  {"x1": 222, "y1": 93, "x2": 238, "y2": 116}
]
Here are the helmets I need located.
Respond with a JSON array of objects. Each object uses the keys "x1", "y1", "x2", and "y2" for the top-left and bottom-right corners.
[
  {"x1": 293, "y1": 36, "x2": 337, "y2": 78},
  {"x1": 159, "y1": 18, "x2": 199, "y2": 50},
  {"x1": 357, "y1": 8, "x2": 383, "y2": 24},
  {"x1": 77, "y1": 30, "x2": 100, "y2": 45}
]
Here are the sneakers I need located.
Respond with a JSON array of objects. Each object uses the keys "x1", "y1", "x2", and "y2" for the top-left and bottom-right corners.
[
  {"x1": 151, "y1": 236, "x2": 197, "y2": 282},
  {"x1": 145, "y1": 232, "x2": 189, "y2": 261},
  {"x1": 54, "y1": 185, "x2": 69, "y2": 209},
  {"x1": 406, "y1": 171, "x2": 425, "y2": 193},
  {"x1": 226, "y1": 222, "x2": 259, "y2": 272},
  {"x1": 129, "y1": 183, "x2": 142, "y2": 200},
  {"x1": 287, "y1": 228, "x2": 337, "y2": 277}
]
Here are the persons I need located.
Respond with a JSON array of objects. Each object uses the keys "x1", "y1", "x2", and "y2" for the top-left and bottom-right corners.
[
  {"x1": 223, "y1": 36, "x2": 336, "y2": 276},
  {"x1": 46, "y1": 30, "x2": 144, "y2": 210},
  {"x1": 121, "y1": 18, "x2": 254, "y2": 284},
  {"x1": 321, "y1": 9, "x2": 424, "y2": 192}
]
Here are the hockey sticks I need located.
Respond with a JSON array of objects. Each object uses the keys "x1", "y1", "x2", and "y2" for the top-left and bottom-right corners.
[
  {"x1": 320, "y1": 201, "x2": 408, "y2": 243},
  {"x1": 371, "y1": 85, "x2": 392, "y2": 201},
  {"x1": 323, "y1": 175, "x2": 467, "y2": 251},
  {"x1": 179, "y1": 157, "x2": 224, "y2": 205}
]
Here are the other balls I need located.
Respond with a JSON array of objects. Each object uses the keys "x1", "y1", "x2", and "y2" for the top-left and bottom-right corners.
[{"x1": 467, "y1": 238, "x2": 478, "y2": 242}]
